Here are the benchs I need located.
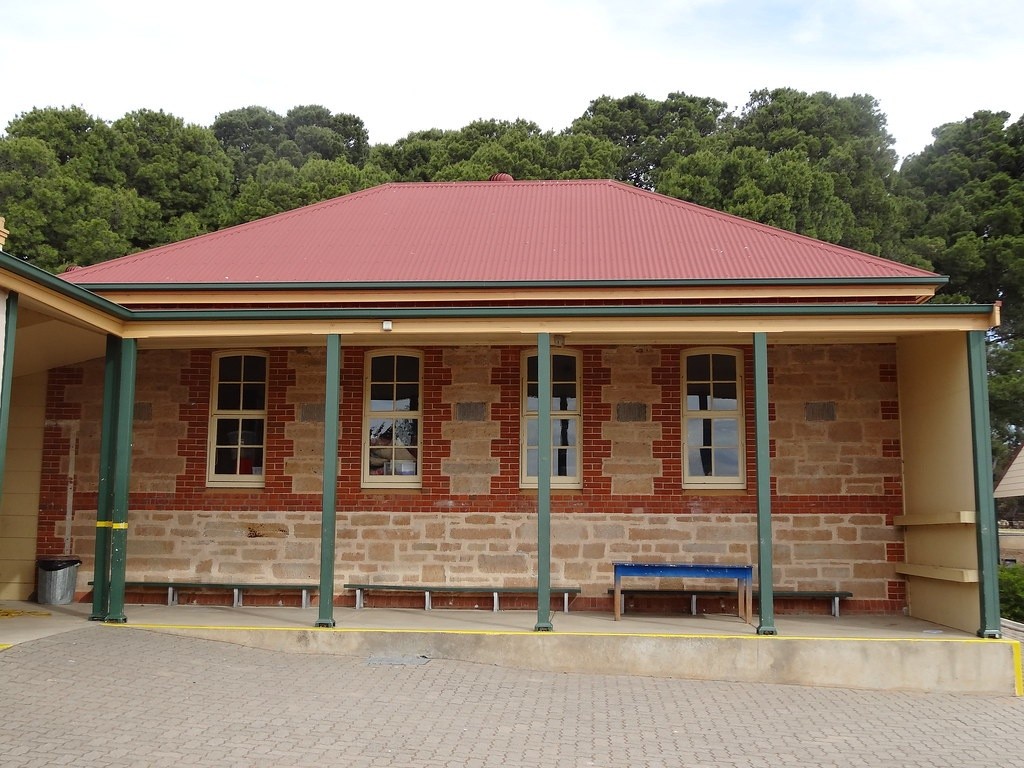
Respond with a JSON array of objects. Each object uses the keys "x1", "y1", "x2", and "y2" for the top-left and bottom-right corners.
[
  {"x1": 607, "y1": 588, "x2": 735, "y2": 616},
  {"x1": 343, "y1": 583, "x2": 581, "y2": 614},
  {"x1": 87, "y1": 579, "x2": 319, "y2": 610},
  {"x1": 752, "y1": 591, "x2": 853, "y2": 618}
]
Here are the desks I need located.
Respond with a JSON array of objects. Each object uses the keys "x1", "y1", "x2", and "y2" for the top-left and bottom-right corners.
[{"x1": 613, "y1": 561, "x2": 754, "y2": 624}]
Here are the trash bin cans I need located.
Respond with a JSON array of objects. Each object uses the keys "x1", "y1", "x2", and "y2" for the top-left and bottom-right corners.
[{"x1": 35, "y1": 555, "x2": 81, "y2": 605}]
